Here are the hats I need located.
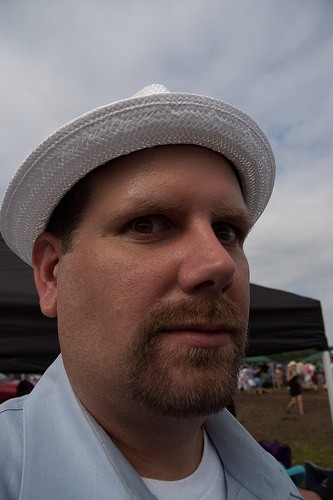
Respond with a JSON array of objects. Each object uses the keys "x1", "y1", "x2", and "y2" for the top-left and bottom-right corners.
[
  {"x1": 0, "y1": 82, "x2": 279, "y2": 269},
  {"x1": 287, "y1": 360, "x2": 297, "y2": 368}
]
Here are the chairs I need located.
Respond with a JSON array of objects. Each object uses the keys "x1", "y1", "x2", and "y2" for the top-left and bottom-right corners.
[{"x1": 286, "y1": 461, "x2": 333, "y2": 500}]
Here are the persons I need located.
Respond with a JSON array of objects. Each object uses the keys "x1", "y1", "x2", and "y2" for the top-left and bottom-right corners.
[
  {"x1": 0, "y1": 83, "x2": 304, "y2": 500},
  {"x1": 297, "y1": 358, "x2": 327, "y2": 391},
  {"x1": 286, "y1": 361, "x2": 305, "y2": 417},
  {"x1": 237, "y1": 363, "x2": 283, "y2": 396}
]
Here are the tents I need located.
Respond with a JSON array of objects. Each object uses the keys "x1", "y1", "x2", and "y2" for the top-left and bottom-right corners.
[{"x1": 0, "y1": 226, "x2": 333, "y2": 422}]
